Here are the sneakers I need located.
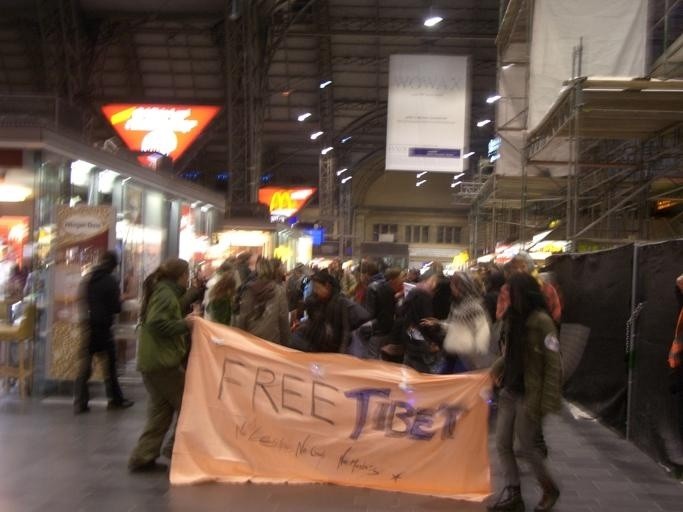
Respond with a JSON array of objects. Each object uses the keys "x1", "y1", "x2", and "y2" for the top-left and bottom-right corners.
[
  {"x1": 108, "y1": 398, "x2": 134, "y2": 410},
  {"x1": 134, "y1": 460, "x2": 167, "y2": 473},
  {"x1": 76, "y1": 407, "x2": 91, "y2": 412}
]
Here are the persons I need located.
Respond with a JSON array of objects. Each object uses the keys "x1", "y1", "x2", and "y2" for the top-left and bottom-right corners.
[
  {"x1": 191, "y1": 249, "x2": 565, "y2": 367},
  {"x1": 67, "y1": 247, "x2": 134, "y2": 415},
  {"x1": 489, "y1": 273, "x2": 560, "y2": 510},
  {"x1": 130, "y1": 257, "x2": 197, "y2": 470}
]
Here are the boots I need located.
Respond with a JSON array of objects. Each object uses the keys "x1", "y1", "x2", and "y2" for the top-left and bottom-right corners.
[
  {"x1": 534, "y1": 474, "x2": 561, "y2": 511},
  {"x1": 487, "y1": 484, "x2": 525, "y2": 511}
]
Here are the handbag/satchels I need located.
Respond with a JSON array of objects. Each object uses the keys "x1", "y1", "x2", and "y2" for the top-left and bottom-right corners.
[{"x1": 335, "y1": 297, "x2": 372, "y2": 331}]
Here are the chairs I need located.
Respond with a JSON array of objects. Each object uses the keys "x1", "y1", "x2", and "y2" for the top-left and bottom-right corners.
[{"x1": 1, "y1": 303, "x2": 39, "y2": 399}]
